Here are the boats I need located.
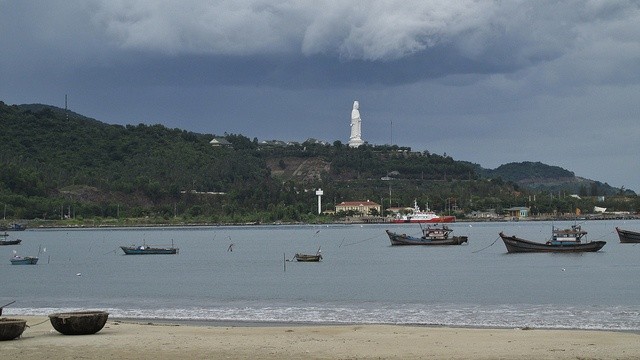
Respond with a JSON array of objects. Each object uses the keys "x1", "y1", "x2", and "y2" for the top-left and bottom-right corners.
[
  {"x1": 10, "y1": 255, "x2": 39, "y2": 265},
  {"x1": 384, "y1": 221, "x2": 469, "y2": 246},
  {"x1": 499, "y1": 218, "x2": 606, "y2": 252},
  {"x1": 0, "y1": 318, "x2": 27, "y2": 340},
  {"x1": 296, "y1": 252, "x2": 321, "y2": 262},
  {"x1": 9, "y1": 224, "x2": 25, "y2": 231},
  {"x1": 1, "y1": 238, "x2": 22, "y2": 246},
  {"x1": 119, "y1": 239, "x2": 179, "y2": 255},
  {"x1": 616, "y1": 227, "x2": 640, "y2": 243},
  {"x1": 0, "y1": 234, "x2": 10, "y2": 236},
  {"x1": 49, "y1": 311, "x2": 109, "y2": 335}
]
